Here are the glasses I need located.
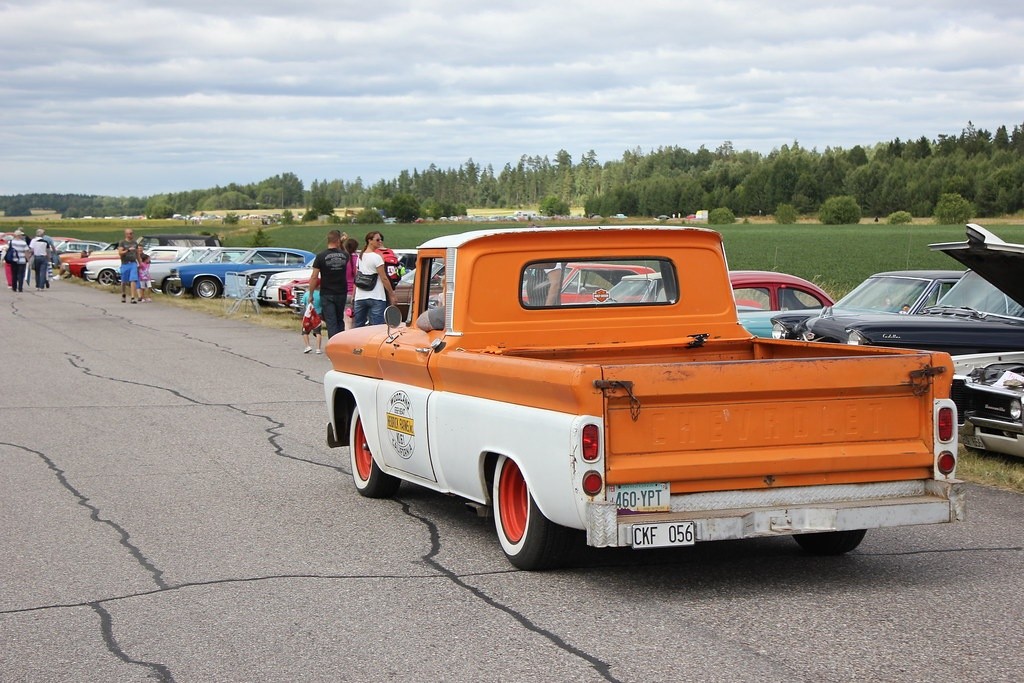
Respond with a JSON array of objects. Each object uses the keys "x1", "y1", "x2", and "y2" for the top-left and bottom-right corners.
[
  {"x1": 125, "y1": 232, "x2": 132, "y2": 235},
  {"x1": 373, "y1": 238, "x2": 381, "y2": 242}
]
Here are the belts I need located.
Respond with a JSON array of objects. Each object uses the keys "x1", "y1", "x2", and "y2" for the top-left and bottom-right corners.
[{"x1": 127, "y1": 260, "x2": 135, "y2": 263}]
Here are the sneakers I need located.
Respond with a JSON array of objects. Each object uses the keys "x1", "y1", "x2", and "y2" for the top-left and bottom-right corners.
[
  {"x1": 304, "y1": 347, "x2": 312, "y2": 353},
  {"x1": 316, "y1": 349, "x2": 322, "y2": 354},
  {"x1": 53, "y1": 274, "x2": 61, "y2": 280}
]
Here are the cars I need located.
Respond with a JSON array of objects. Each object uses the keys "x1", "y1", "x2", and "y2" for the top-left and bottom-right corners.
[
  {"x1": 924, "y1": 223, "x2": 1024, "y2": 458},
  {"x1": 766, "y1": 268, "x2": 1024, "y2": 357},
  {"x1": 0, "y1": 232, "x2": 413, "y2": 315},
  {"x1": 475, "y1": 261, "x2": 832, "y2": 341}
]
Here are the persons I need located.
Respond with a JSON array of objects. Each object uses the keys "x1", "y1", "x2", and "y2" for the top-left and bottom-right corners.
[
  {"x1": 526, "y1": 262, "x2": 562, "y2": 307},
  {"x1": 301, "y1": 230, "x2": 398, "y2": 354},
  {"x1": 417, "y1": 276, "x2": 445, "y2": 333},
  {"x1": 0, "y1": 226, "x2": 60, "y2": 292},
  {"x1": 118, "y1": 228, "x2": 152, "y2": 304}
]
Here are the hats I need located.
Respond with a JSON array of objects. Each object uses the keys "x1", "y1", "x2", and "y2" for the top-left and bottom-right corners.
[{"x1": 14, "y1": 231, "x2": 25, "y2": 235}]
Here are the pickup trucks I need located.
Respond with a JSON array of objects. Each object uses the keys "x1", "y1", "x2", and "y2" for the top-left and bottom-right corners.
[{"x1": 324, "y1": 224, "x2": 961, "y2": 572}]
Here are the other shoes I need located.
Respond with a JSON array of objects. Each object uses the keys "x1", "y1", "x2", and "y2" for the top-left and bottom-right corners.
[
  {"x1": 138, "y1": 297, "x2": 151, "y2": 302},
  {"x1": 8, "y1": 285, "x2": 23, "y2": 293},
  {"x1": 122, "y1": 294, "x2": 137, "y2": 303},
  {"x1": 36, "y1": 280, "x2": 50, "y2": 290}
]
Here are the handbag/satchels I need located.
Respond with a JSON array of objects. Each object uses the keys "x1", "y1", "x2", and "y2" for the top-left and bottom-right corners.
[
  {"x1": 4, "y1": 240, "x2": 19, "y2": 264},
  {"x1": 354, "y1": 259, "x2": 379, "y2": 291},
  {"x1": 303, "y1": 303, "x2": 322, "y2": 333},
  {"x1": 346, "y1": 299, "x2": 354, "y2": 318}
]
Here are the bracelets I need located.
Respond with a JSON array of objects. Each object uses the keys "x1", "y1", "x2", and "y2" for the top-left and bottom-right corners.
[{"x1": 128, "y1": 249, "x2": 130, "y2": 252}]
